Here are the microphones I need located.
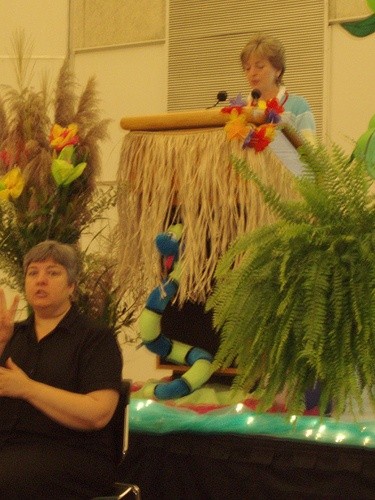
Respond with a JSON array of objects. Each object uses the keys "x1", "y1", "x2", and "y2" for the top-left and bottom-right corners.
[
  {"x1": 206, "y1": 91, "x2": 227, "y2": 109},
  {"x1": 251, "y1": 89, "x2": 261, "y2": 107}
]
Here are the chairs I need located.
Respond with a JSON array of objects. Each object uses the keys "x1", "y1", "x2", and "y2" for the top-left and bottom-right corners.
[{"x1": 93, "y1": 381, "x2": 142, "y2": 500}]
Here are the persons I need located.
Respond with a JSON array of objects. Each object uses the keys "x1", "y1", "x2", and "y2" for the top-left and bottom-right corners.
[
  {"x1": 0, "y1": 240, "x2": 123, "y2": 500},
  {"x1": 241, "y1": 34, "x2": 317, "y2": 177}
]
